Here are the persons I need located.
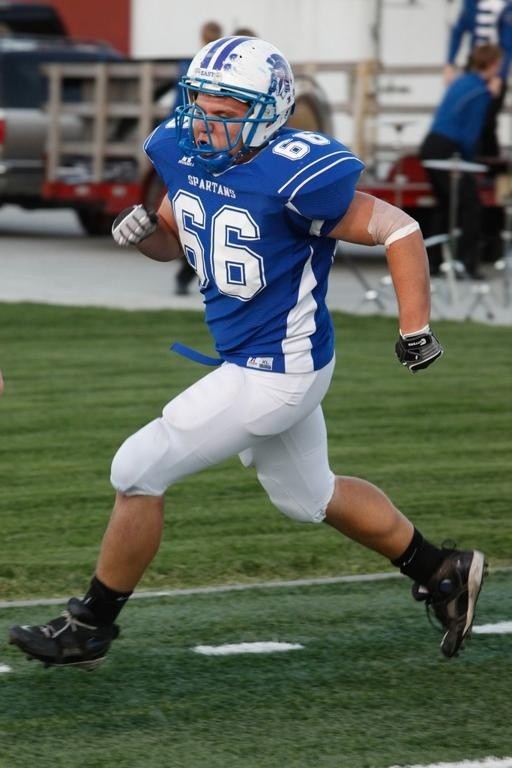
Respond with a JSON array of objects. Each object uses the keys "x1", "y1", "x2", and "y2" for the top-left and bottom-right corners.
[
  {"x1": 9, "y1": 35, "x2": 489, "y2": 671},
  {"x1": 447, "y1": 0, "x2": 477, "y2": 62},
  {"x1": 419, "y1": 45, "x2": 506, "y2": 280},
  {"x1": 498, "y1": 1, "x2": 512, "y2": 76}
]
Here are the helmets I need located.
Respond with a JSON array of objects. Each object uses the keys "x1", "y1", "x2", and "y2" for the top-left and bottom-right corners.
[{"x1": 185, "y1": 36, "x2": 295, "y2": 148}]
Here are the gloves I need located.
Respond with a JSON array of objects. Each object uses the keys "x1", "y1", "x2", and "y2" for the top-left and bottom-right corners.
[
  {"x1": 396, "y1": 325, "x2": 444, "y2": 374},
  {"x1": 111, "y1": 203, "x2": 159, "y2": 247}
]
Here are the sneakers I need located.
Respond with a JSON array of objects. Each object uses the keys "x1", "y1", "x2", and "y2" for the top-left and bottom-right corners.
[
  {"x1": 411, "y1": 550, "x2": 489, "y2": 659},
  {"x1": 6, "y1": 598, "x2": 121, "y2": 672}
]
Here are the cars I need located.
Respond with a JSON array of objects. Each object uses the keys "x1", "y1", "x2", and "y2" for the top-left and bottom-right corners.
[{"x1": 0, "y1": 38, "x2": 333, "y2": 235}]
